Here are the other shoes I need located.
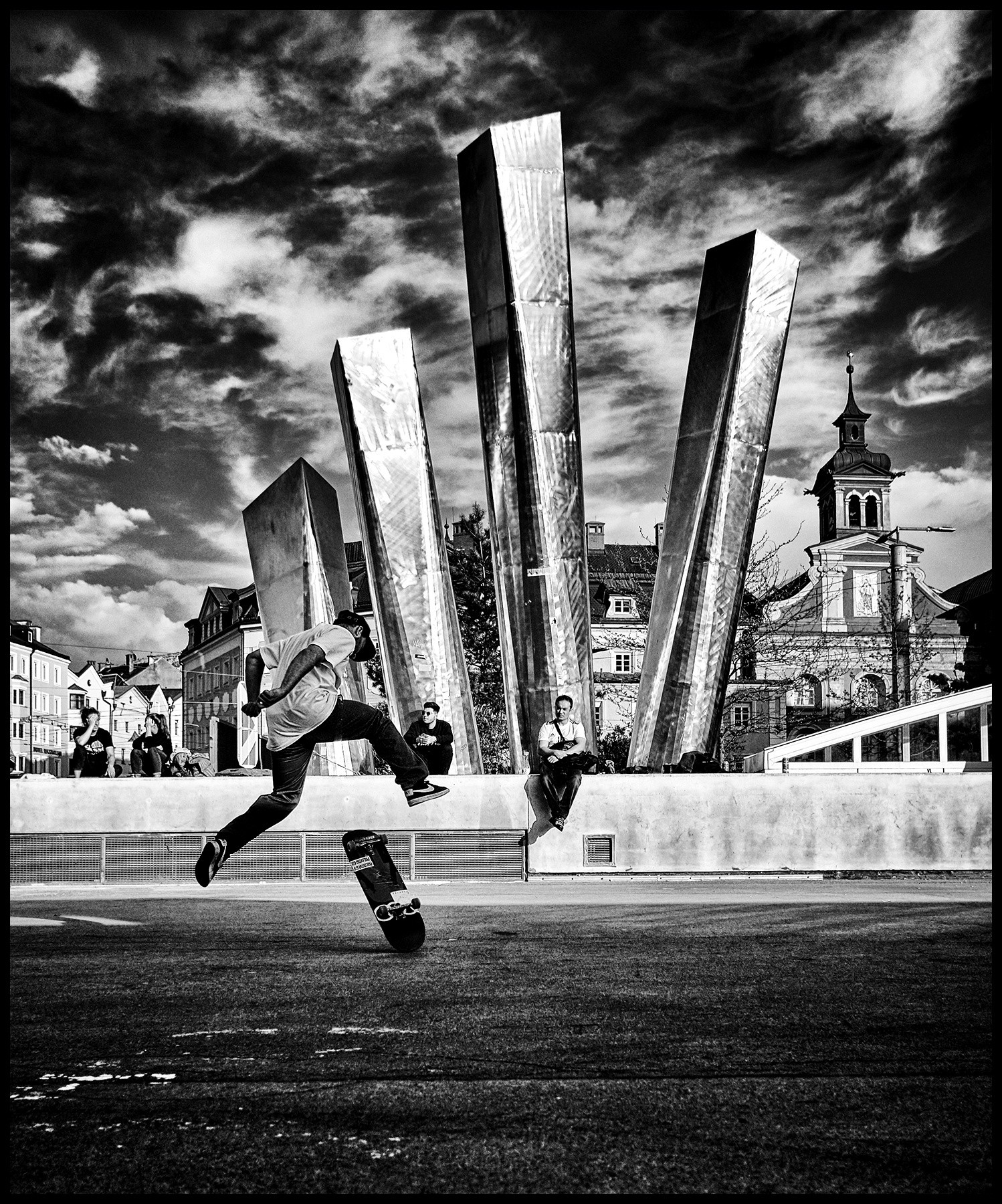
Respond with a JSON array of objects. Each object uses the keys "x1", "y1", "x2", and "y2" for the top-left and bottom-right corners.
[{"x1": 548, "y1": 817, "x2": 565, "y2": 833}]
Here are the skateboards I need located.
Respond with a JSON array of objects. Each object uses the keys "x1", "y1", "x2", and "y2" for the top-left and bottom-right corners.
[{"x1": 341, "y1": 829, "x2": 426, "y2": 954}]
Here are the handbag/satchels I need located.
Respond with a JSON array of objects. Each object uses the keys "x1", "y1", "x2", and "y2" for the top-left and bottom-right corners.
[
  {"x1": 542, "y1": 740, "x2": 578, "y2": 772},
  {"x1": 167, "y1": 752, "x2": 215, "y2": 777}
]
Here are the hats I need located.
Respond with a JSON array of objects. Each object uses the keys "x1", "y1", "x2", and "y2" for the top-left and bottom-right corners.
[{"x1": 333, "y1": 611, "x2": 376, "y2": 662}]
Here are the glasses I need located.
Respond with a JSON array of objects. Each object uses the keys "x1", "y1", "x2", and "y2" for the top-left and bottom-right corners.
[{"x1": 422, "y1": 710, "x2": 434, "y2": 716}]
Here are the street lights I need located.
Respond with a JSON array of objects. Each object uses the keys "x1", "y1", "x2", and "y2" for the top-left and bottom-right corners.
[{"x1": 873, "y1": 525, "x2": 956, "y2": 708}]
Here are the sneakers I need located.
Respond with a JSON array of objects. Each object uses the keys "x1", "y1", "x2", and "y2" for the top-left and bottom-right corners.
[
  {"x1": 404, "y1": 781, "x2": 450, "y2": 807},
  {"x1": 195, "y1": 838, "x2": 230, "y2": 888}
]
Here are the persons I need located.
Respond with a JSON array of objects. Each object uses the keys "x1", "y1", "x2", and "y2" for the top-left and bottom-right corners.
[
  {"x1": 72, "y1": 707, "x2": 123, "y2": 778},
  {"x1": 195, "y1": 610, "x2": 450, "y2": 888},
  {"x1": 403, "y1": 702, "x2": 453, "y2": 775},
  {"x1": 131, "y1": 713, "x2": 173, "y2": 777},
  {"x1": 538, "y1": 695, "x2": 587, "y2": 831}
]
